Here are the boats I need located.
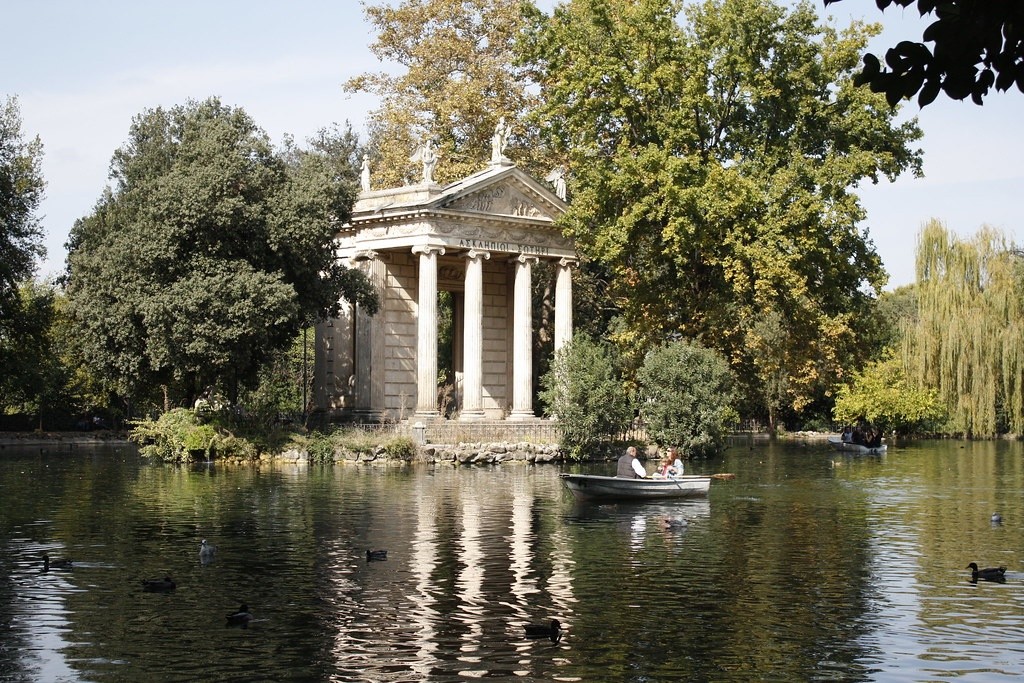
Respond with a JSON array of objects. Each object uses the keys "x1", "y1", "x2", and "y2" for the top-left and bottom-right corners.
[
  {"x1": 827, "y1": 438, "x2": 888, "y2": 453},
  {"x1": 557, "y1": 471, "x2": 711, "y2": 500}
]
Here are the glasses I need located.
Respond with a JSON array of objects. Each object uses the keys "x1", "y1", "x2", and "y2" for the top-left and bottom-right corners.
[{"x1": 666, "y1": 450, "x2": 671, "y2": 452}]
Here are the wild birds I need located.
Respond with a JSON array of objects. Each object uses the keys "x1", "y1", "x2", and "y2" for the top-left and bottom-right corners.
[
  {"x1": 990, "y1": 512, "x2": 1002, "y2": 528},
  {"x1": 140, "y1": 576, "x2": 177, "y2": 594},
  {"x1": 225, "y1": 604, "x2": 250, "y2": 625},
  {"x1": 964, "y1": 562, "x2": 1008, "y2": 583},
  {"x1": 599, "y1": 504, "x2": 619, "y2": 513},
  {"x1": 365, "y1": 549, "x2": 388, "y2": 563},
  {"x1": 41, "y1": 554, "x2": 74, "y2": 571},
  {"x1": 832, "y1": 460, "x2": 842, "y2": 469},
  {"x1": 522, "y1": 619, "x2": 563, "y2": 647},
  {"x1": 664, "y1": 519, "x2": 688, "y2": 527},
  {"x1": 199, "y1": 539, "x2": 221, "y2": 556},
  {"x1": 960, "y1": 446, "x2": 965, "y2": 449}
]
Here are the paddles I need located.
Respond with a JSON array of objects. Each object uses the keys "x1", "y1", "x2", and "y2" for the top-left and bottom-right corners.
[{"x1": 646, "y1": 473, "x2": 734, "y2": 479}]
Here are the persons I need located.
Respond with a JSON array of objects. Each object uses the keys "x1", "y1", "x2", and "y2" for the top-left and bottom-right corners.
[
  {"x1": 617, "y1": 447, "x2": 647, "y2": 480},
  {"x1": 652, "y1": 447, "x2": 683, "y2": 480},
  {"x1": 495, "y1": 116, "x2": 512, "y2": 154},
  {"x1": 422, "y1": 141, "x2": 442, "y2": 182},
  {"x1": 841, "y1": 426, "x2": 881, "y2": 449},
  {"x1": 360, "y1": 154, "x2": 372, "y2": 192},
  {"x1": 556, "y1": 165, "x2": 567, "y2": 202}
]
